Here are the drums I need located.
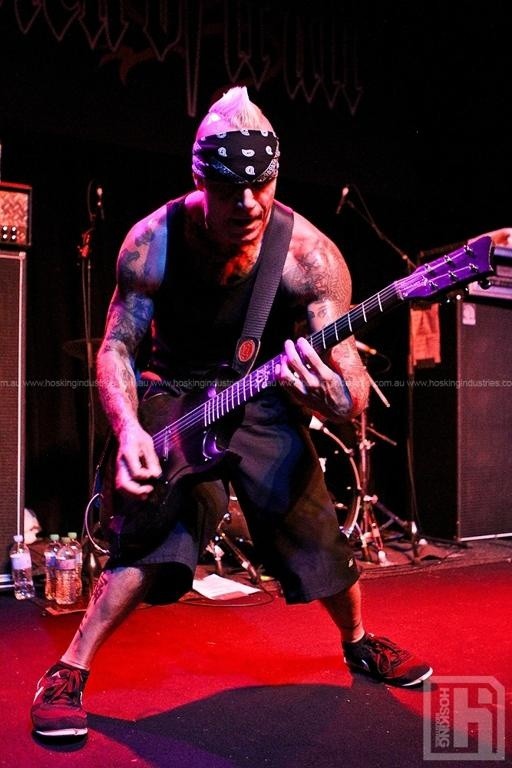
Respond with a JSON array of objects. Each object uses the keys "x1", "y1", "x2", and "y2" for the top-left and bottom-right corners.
[{"x1": 220, "y1": 416, "x2": 361, "y2": 542}]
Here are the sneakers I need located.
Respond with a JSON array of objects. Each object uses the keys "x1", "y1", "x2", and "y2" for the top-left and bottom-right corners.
[
  {"x1": 30, "y1": 663, "x2": 89, "y2": 738},
  {"x1": 341, "y1": 632, "x2": 433, "y2": 687}
]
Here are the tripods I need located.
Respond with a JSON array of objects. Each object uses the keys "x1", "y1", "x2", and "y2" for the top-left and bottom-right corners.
[
  {"x1": 75, "y1": 217, "x2": 115, "y2": 597},
  {"x1": 339, "y1": 194, "x2": 474, "y2": 566}
]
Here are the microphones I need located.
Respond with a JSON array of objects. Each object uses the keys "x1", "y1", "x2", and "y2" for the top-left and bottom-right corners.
[{"x1": 335, "y1": 184, "x2": 351, "y2": 217}]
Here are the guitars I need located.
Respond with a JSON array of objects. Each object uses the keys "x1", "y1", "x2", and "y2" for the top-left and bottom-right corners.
[{"x1": 102, "y1": 236, "x2": 497, "y2": 554}]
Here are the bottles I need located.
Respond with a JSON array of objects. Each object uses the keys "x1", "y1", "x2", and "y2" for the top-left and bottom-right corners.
[
  {"x1": 43, "y1": 532, "x2": 83, "y2": 606},
  {"x1": 10, "y1": 536, "x2": 35, "y2": 601}
]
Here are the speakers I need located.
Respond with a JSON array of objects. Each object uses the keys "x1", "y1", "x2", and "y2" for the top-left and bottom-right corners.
[
  {"x1": 1, "y1": 250, "x2": 29, "y2": 594},
  {"x1": 403, "y1": 293, "x2": 511, "y2": 547}
]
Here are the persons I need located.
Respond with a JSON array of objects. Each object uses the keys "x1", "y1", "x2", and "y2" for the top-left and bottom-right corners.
[{"x1": 29, "y1": 85, "x2": 432, "y2": 740}]
